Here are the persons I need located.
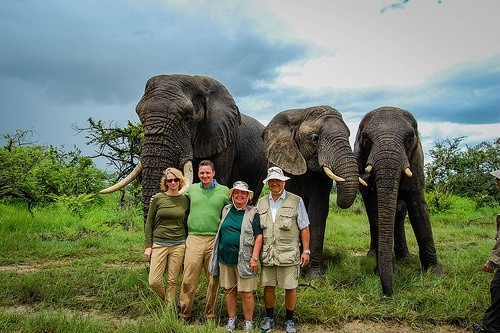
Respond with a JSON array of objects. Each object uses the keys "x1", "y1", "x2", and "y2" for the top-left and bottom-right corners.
[
  {"x1": 144, "y1": 167, "x2": 191, "y2": 314},
  {"x1": 473, "y1": 170, "x2": 500, "y2": 333},
  {"x1": 149, "y1": 160, "x2": 259, "y2": 325},
  {"x1": 209, "y1": 181, "x2": 263, "y2": 333},
  {"x1": 257, "y1": 167, "x2": 311, "y2": 333}
]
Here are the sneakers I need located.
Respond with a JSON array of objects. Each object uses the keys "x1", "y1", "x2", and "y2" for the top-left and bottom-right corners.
[
  {"x1": 243, "y1": 320, "x2": 255, "y2": 333},
  {"x1": 284, "y1": 320, "x2": 297, "y2": 333},
  {"x1": 207, "y1": 318, "x2": 216, "y2": 329},
  {"x1": 225, "y1": 316, "x2": 238, "y2": 333},
  {"x1": 260, "y1": 317, "x2": 275, "y2": 333}
]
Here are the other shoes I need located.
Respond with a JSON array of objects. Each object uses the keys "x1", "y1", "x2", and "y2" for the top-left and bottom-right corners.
[{"x1": 177, "y1": 316, "x2": 193, "y2": 325}]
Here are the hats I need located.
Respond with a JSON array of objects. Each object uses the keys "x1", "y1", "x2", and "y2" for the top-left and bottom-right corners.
[
  {"x1": 262, "y1": 167, "x2": 290, "y2": 185},
  {"x1": 228, "y1": 181, "x2": 254, "y2": 201}
]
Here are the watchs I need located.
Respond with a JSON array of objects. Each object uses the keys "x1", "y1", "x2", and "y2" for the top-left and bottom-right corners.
[
  {"x1": 303, "y1": 250, "x2": 310, "y2": 254},
  {"x1": 252, "y1": 256, "x2": 259, "y2": 261}
]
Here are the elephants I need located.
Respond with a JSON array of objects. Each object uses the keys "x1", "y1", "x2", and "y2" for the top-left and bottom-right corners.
[
  {"x1": 262, "y1": 105, "x2": 358, "y2": 281},
  {"x1": 354, "y1": 107, "x2": 444, "y2": 297},
  {"x1": 100, "y1": 74, "x2": 268, "y2": 234}
]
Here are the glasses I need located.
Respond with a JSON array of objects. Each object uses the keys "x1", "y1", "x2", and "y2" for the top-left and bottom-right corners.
[{"x1": 166, "y1": 178, "x2": 180, "y2": 183}]
